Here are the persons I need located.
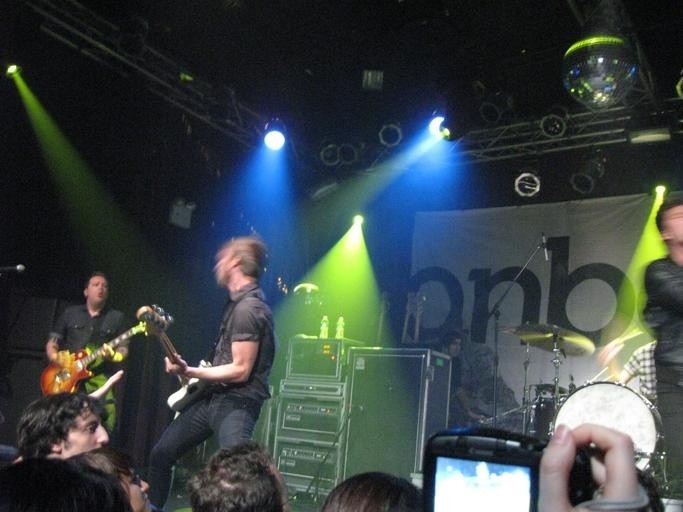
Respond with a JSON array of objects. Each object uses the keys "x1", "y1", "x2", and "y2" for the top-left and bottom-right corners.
[
  {"x1": 426, "y1": 330, "x2": 486, "y2": 422},
  {"x1": 0, "y1": 390, "x2": 153, "y2": 512},
  {"x1": 185, "y1": 439, "x2": 290, "y2": 512},
  {"x1": 318, "y1": 470, "x2": 424, "y2": 512},
  {"x1": 44, "y1": 271, "x2": 131, "y2": 433},
  {"x1": 146, "y1": 236, "x2": 276, "y2": 512},
  {"x1": 639, "y1": 196, "x2": 683, "y2": 501},
  {"x1": 536, "y1": 423, "x2": 654, "y2": 512}
]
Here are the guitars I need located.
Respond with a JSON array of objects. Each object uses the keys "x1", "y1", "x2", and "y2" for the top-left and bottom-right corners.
[
  {"x1": 39, "y1": 303, "x2": 172, "y2": 397},
  {"x1": 136, "y1": 305, "x2": 213, "y2": 412}
]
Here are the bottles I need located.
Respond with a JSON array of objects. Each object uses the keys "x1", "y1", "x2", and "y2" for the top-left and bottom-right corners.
[
  {"x1": 335, "y1": 315, "x2": 344, "y2": 340},
  {"x1": 319, "y1": 314, "x2": 329, "y2": 339}
]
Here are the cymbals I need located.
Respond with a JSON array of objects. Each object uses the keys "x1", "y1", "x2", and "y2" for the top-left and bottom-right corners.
[{"x1": 516, "y1": 324, "x2": 596, "y2": 358}]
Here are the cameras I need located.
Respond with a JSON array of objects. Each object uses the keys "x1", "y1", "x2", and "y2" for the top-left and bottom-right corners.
[{"x1": 423, "y1": 426, "x2": 593, "y2": 512}]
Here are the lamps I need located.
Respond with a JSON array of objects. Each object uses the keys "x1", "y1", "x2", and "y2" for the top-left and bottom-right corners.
[{"x1": 256, "y1": 73, "x2": 608, "y2": 200}]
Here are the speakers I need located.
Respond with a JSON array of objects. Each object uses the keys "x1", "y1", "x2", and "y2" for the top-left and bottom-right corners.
[{"x1": 340, "y1": 346, "x2": 453, "y2": 490}]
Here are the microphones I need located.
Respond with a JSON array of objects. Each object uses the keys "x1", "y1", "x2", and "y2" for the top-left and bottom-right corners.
[
  {"x1": 0, "y1": 264, "x2": 26, "y2": 275},
  {"x1": 541, "y1": 233, "x2": 550, "y2": 261}
]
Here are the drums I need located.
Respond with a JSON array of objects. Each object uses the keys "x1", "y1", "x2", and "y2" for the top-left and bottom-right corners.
[{"x1": 552, "y1": 380, "x2": 664, "y2": 471}]
